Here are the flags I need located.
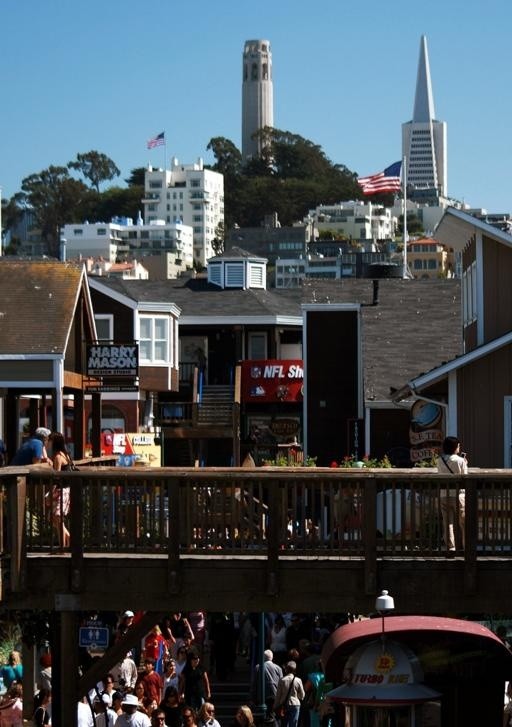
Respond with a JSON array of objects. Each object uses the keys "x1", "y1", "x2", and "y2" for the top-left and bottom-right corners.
[
  {"x1": 356, "y1": 159, "x2": 401, "y2": 195},
  {"x1": 146, "y1": 131, "x2": 166, "y2": 148}
]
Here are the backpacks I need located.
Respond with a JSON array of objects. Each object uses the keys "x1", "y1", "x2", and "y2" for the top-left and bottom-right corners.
[{"x1": 23, "y1": 707, "x2": 46, "y2": 727}]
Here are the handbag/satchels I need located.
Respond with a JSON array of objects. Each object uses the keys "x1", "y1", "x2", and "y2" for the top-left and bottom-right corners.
[
  {"x1": 302, "y1": 687, "x2": 317, "y2": 709},
  {"x1": 276, "y1": 702, "x2": 289, "y2": 719},
  {"x1": 155, "y1": 659, "x2": 162, "y2": 673},
  {"x1": 319, "y1": 702, "x2": 334, "y2": 717}
]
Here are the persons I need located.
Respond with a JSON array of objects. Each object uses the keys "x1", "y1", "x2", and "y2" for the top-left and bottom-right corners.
[
  {"x1": 493, "y1": 621, "x2": 511, "y2": 650},
  {"x1": 503, "y1": 680, "x2": 512, "y2": 726},
  {"x1": 76, "y1": 610, "x2": 346, "y2": 726},
  {"x1": 0, "y1": 651, "x2": 52, "y2": 726},
  {"x1": 437, "y1": 437, "x2": 475, "y2": 550},
  {"x1": 0, "y1": 426, "x2": 76, "y2": 546}
]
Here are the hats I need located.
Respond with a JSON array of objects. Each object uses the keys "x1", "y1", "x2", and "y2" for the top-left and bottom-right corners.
[
  {"x1": 121, "y1": 694, "x2": 138, "y2": 706},
  {"x1": 94, "y1": 693, "x2": 110, "y2": 704},
  {"x1": 36, "y1": 427, "x2": 51, "y2": 437},
  {"x1": 0, "y1": 695, "x2": 16, "y2": 709},
  {"x1": 40, "y1": 653, "x2": 52, "y2": 667},
  {"x1": 125, "y1": 610, "x2": 134, "y2": 617}
]
